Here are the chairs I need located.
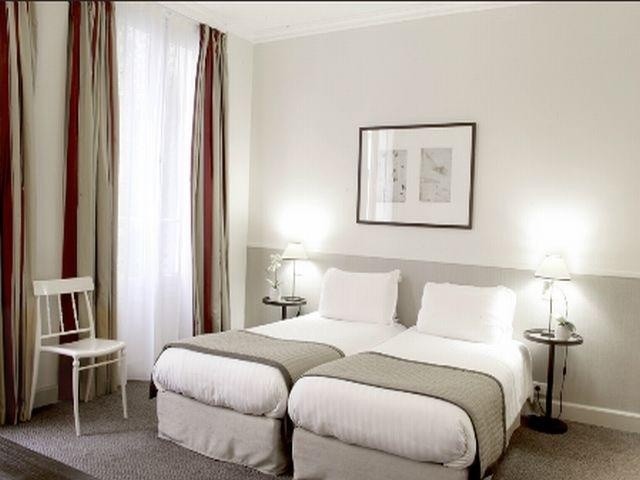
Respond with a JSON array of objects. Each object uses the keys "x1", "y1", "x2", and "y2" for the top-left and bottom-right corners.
[{"x1": 25, "y1": 275, "x2": 127, "y2": 436}]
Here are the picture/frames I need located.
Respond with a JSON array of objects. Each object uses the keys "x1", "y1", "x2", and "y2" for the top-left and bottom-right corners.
[{"x1": 356, "y1": 122, "x2": 476, "y2": 230}]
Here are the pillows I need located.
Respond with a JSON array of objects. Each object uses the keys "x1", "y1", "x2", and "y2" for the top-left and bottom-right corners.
[
  {"x1": 417, "y1": 282, "x2": 514, "y2": 344},
  {"x1": 318, "y1": 263, "x2": 401, "y2": 327}
]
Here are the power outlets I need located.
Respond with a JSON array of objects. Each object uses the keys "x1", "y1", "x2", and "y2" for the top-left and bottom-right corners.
[{"x1": 533, "y1": 381, "x2": 547, "y2": 395}]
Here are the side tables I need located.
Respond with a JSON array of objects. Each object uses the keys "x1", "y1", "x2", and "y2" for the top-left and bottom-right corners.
[
  {"x1": 263, "y1": 296, "x2": 306, "y2": 320},
  {"x1": 524, "y1": 327, "x2": 583, "y2": 434}
]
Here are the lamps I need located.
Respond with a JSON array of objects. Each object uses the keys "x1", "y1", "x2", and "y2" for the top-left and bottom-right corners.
[
  {"x1": 535, "y1": 258, "x2": 571, "y2": 339},
  {"x1": 281, "y1": 241, "x2": 309, "y2": 301}
]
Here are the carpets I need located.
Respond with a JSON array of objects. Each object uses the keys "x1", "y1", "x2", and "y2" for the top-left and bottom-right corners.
[{"x1": 0, "y1": 436, "x2": 93, "y2": 480}]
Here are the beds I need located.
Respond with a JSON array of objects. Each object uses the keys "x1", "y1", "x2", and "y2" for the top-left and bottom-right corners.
[
  {"x1": 287, "y1": 327, "x2": 533, "y2": 480},
  {"x1": 148, "y1": 310, "x2": 408, "y2": 477}
]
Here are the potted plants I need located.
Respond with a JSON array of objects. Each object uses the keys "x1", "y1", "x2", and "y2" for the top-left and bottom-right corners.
[
  {"x1": 265, "y1": 254, "x2": 284, "y2": 302},
  {"x1": 554, "y1": 317, "x2": 574, "y2": 338}
]
